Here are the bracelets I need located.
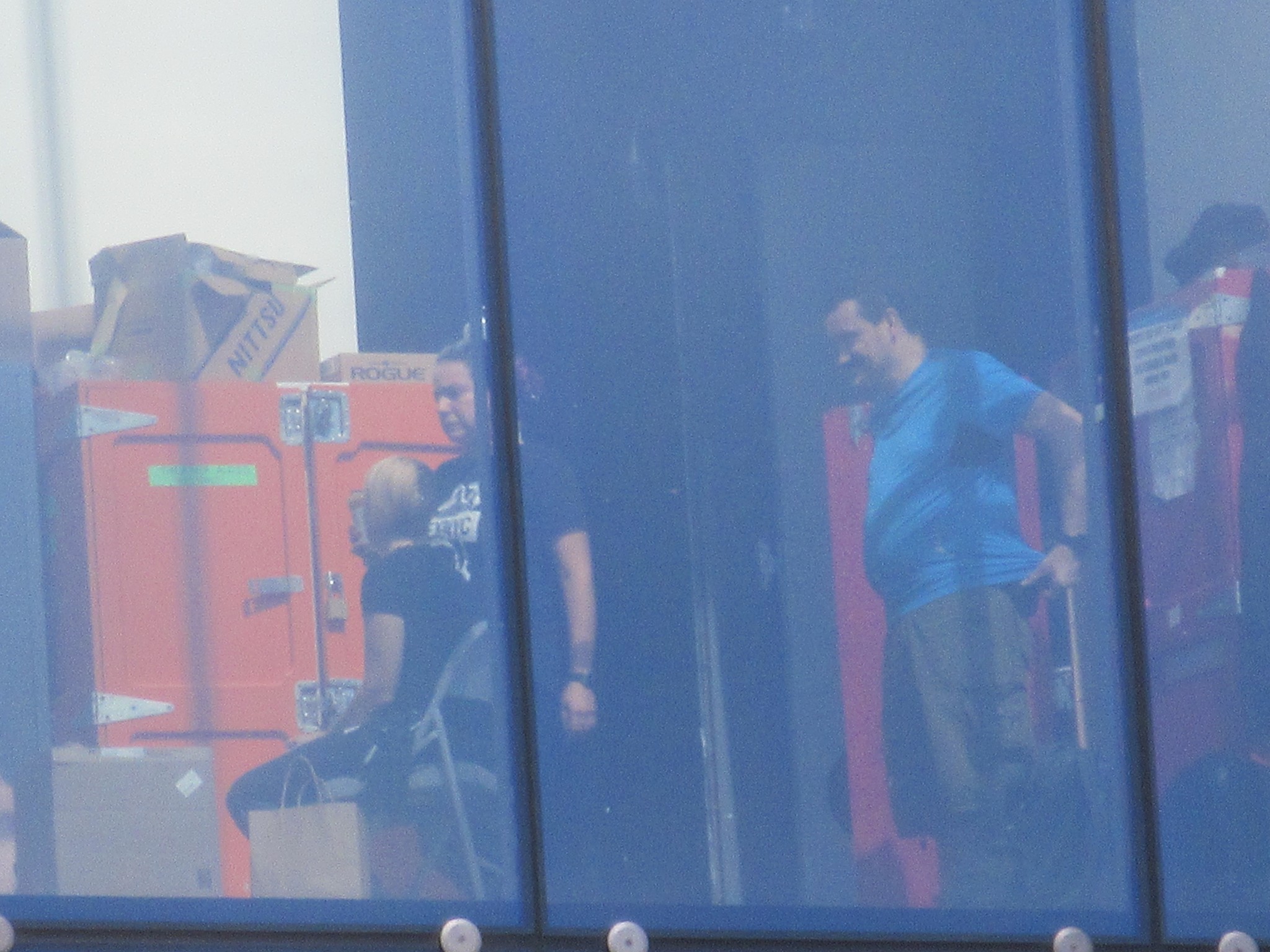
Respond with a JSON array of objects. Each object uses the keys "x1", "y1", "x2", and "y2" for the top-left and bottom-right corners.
[{"x1": 568, "y1": 669, "x2": 599, "y2": 694}]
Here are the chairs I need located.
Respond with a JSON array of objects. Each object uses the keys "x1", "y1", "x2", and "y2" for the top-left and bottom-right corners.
[{"x1": 322, "y1": 617, "x2": 496, "y2": 899}]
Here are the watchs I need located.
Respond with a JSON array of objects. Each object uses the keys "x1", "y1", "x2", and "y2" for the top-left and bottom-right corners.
[{"x1": 1059, "y1": 528, "x2": 1091, "y2": 560}]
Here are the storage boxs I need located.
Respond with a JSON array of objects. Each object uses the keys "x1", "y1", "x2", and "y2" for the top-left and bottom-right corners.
[{"x1": 88, "y1": 228, "x2": 337, "y2": 385}]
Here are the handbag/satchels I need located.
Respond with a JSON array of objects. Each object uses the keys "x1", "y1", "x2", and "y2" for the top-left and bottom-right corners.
[{"x1": 249, "y1": 757, "x2": 372, "y2": 898}]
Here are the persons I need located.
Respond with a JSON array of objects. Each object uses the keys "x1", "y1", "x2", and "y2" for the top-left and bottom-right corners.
[
  {"x1": 824, "y1": 286, "x2": 1091, "y2": 913},
  {"x1": 344, "y1": 339, "x2": 599, "y2": 905},
  {"x1": 227, "y1": 453, "x2": 499, "y2": 898}
]
